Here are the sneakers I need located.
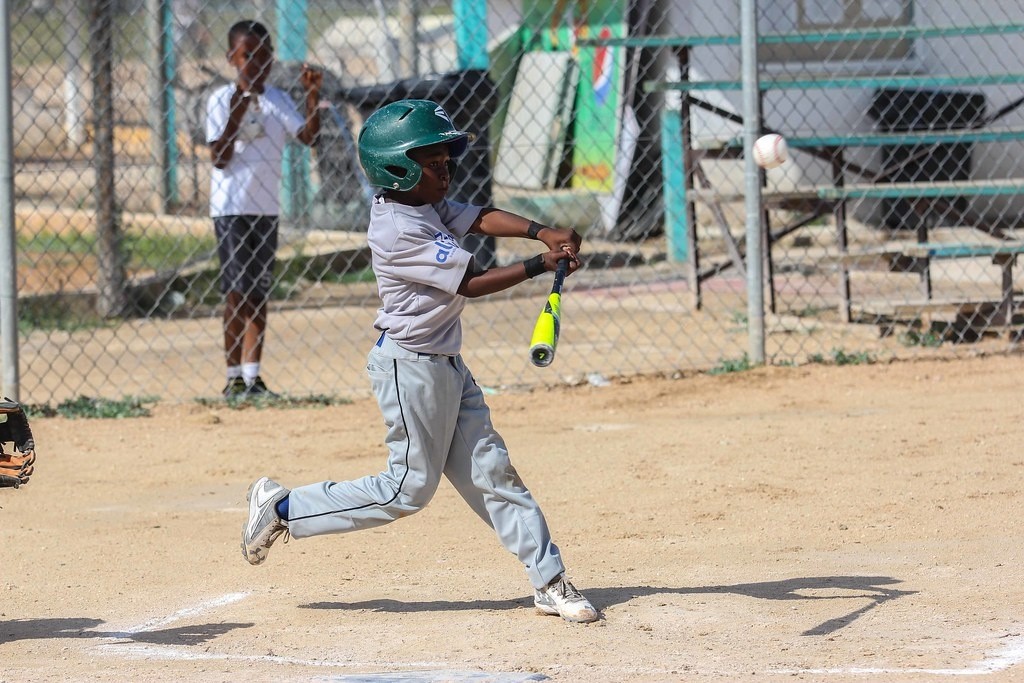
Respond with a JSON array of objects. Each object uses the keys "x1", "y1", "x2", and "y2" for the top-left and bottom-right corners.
[
  {"x1": 240, "y1": 476, "x2": 291, "y2": 566},
  {"x1": 534, "y1": 572, "x2": 598, "y2": 623}
]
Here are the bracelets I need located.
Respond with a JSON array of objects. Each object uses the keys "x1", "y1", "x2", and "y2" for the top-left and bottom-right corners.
[
  {"x1": 526, "y1": 220, "x2": 549, "y2": 240},
  {"x1": 524, "y1": 253, "x2": 548, "y2": 279}
]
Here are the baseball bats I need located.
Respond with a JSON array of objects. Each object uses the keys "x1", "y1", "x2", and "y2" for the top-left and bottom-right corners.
[{"x1": 527, "y1": 255, "x2": 568, "y2": 368}]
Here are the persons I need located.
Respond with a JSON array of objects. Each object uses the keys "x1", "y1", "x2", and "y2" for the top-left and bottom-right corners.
[
  {"x1": 207, "y1": 21, "x2": 323, "y2": 404},
  {"x1": 240, "y1": 99, "x2": 600, "y2": 623}
]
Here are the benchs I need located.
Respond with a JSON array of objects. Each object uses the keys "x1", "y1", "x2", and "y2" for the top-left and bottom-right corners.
[{"x1": 574, "y1": 20, "x2": 1024, "y2": 307}]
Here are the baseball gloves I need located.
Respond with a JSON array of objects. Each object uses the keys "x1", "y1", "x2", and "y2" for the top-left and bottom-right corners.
[{"x1": 0, "y1": 396, "x2": 36, "y2": 490}]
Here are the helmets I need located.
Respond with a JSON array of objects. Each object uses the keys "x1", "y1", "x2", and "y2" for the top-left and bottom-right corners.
[{"x1": 358, "y1": 99, "x2": 469, "y2": 191}]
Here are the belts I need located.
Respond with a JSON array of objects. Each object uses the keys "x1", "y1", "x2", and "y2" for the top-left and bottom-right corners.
[{"x1": 377, "y1": 333, "x2": 435, "y2": 356}]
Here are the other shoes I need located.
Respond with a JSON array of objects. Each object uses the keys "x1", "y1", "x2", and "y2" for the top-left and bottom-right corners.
[
  {"x1": 224, "y1": 375, "x2": 246, "y2": 399},
  {"x1": 249, "y1": 376, "x2": 277, "y2": 399}
]
